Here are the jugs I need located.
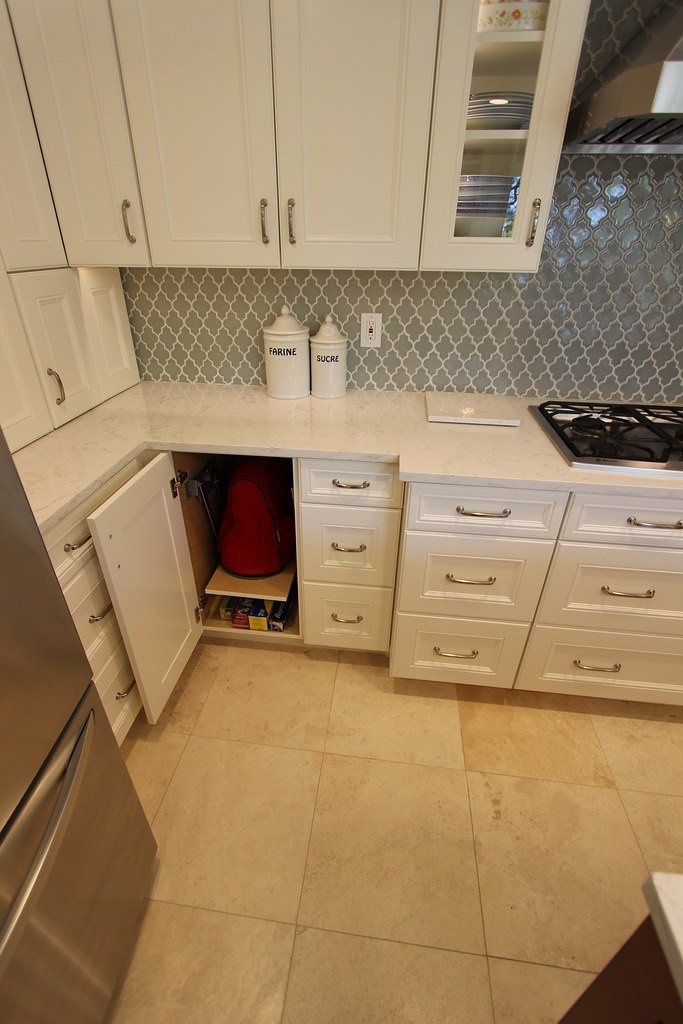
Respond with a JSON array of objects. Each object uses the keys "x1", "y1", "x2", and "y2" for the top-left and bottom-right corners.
[
  {"x1": 310, "y1": 315, "x2": 349, "y2": 399},
  {"x1": 261, "y1": 303, "x2": 311, "y2": 399}
]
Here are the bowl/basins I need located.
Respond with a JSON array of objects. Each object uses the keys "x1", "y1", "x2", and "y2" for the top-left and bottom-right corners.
[{"x1": 456, "y1": 170, "x2": 513, "y2": 238}]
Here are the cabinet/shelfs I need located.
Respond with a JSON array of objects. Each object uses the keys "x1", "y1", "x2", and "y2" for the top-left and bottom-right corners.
[
  {"x1": 513, "y1": 491, "x2": 682, "y2": 710},
  {"x1": 0, "y1": 265, "x2": 142, "y2": 454},
  {"x1": 109, "y1": 0, "x2": 443, "y2": 270},
  {"x1": 414, "y1": 0, "x2": 592, "y2": 274},
  {"x1": 0, "y1": 0, "x2": 155, "y2": 271},
  {"x1": 387, "y1": 475, "x2": 576, "y2": 692},
  {"x1": 85, "y1": 441, "x2": 408, "y2": 728},
  {"x1": 42, "y1": 457, "x2": 144, "y2": 748}
]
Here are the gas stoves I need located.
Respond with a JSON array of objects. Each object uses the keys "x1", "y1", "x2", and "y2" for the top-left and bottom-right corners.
[{"x1": 527, "y1": 394, "x2": 683, "y2": 476}]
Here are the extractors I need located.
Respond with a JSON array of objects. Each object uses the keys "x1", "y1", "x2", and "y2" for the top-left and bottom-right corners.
[{"x1": 566, "y1": 2, "x2": 683, "y2": 154}]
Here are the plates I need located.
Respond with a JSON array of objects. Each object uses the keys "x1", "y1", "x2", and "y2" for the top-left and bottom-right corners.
[{"x1": 465, "y1": 90, "x2": 536, "y2": 129}]
